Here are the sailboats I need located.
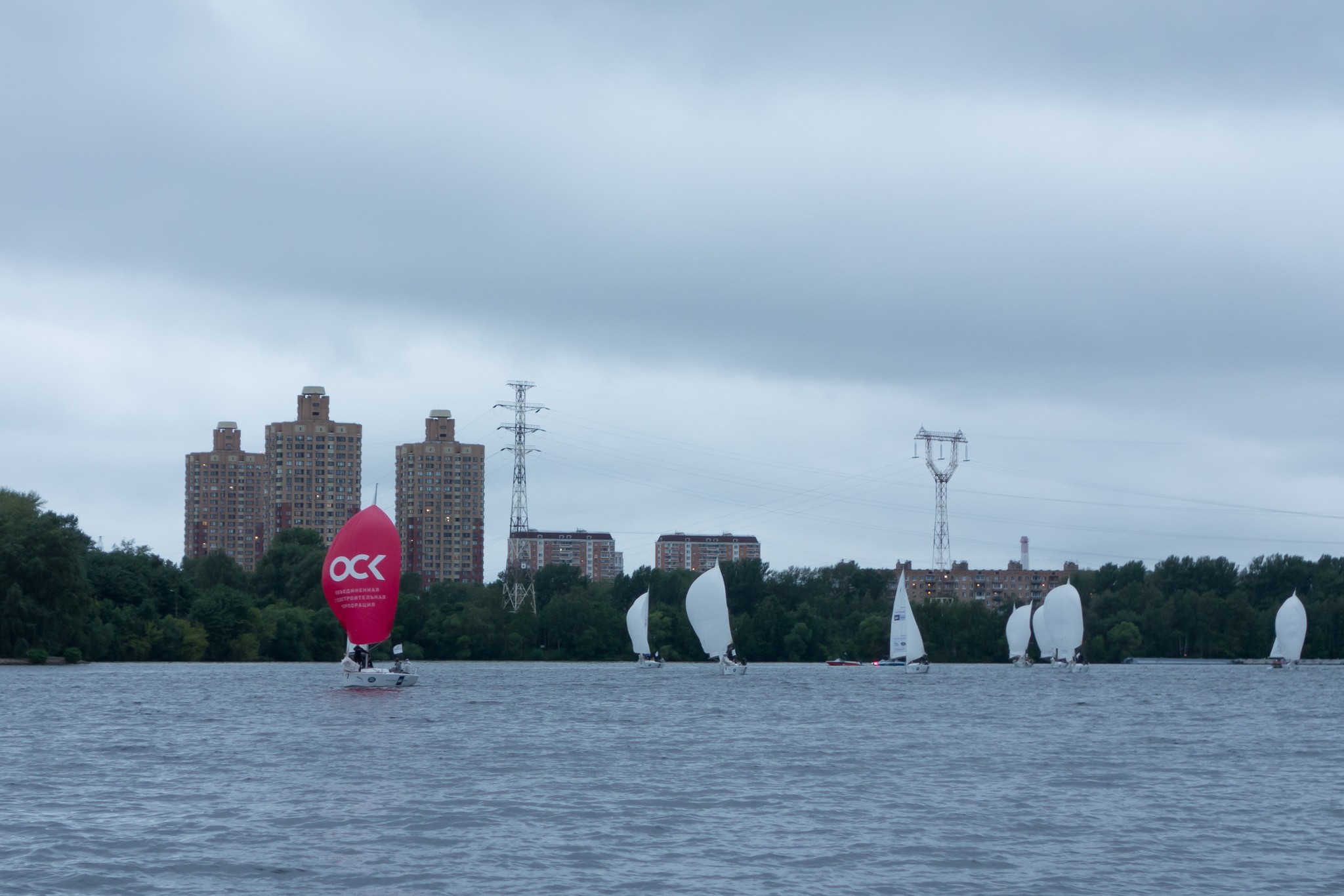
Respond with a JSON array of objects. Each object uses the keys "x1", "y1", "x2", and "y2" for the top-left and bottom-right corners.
[
  {"x1": 321, "y1": 483, "x2": 420, "y2": 690},
  {"x1": 1266, "y1": 586, "x2": 1307, "y2": 671},
  {"x1": 1043, "y1": 576, "x2": 1090, "y2": 673},
  {"x1": 872, "y1": 565, "x2": 908, "y2": 667},
  {"x1": 685, "y1": 556, "x2": 748, "y2": 675},
  {"x1": 1031, "y1": 605, "x2": 1067, "y2": 668},
  {"x1": 626, "y1": 585, "x2": 667, "y2": 669},
  {"x1": 905, "y1": 595, "x2": 931, "y2": 673},
  {"x1": 1004, "y1": 599, "x2": 1034, "y2": 668}
]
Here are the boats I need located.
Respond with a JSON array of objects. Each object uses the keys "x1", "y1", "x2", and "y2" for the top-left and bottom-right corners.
[{"x1": 825, "y1": 657, "x2": 864, "y2": 666}]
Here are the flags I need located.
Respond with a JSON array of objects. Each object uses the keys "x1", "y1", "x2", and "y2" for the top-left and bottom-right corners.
[{"x1": 393, "y1": 644, "x2": 403, "y2": 654}]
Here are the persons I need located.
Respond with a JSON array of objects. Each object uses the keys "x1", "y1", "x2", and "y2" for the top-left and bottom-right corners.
[
  {"x1": 402, "y1": 658, "x2": 412, "y2": 674},
  {"x1": 726, "y1": 646, "x2": 747, "y2": 666},
  {"x1": 353, "y1": 643, "x2": 373, "y2": 671},
  {"x1": 1072, "y1": 653, "x2": 1089, "y2": 666},
  {"x1": 644, "y1": 650, "x2": 665, "y2": 663},
  {"x1": 1012, "y1": 653, "x2": 1034, "y2": 665},
  {"x1": 389, "y1": 657, "x2": 402, "y2": 674}
]
